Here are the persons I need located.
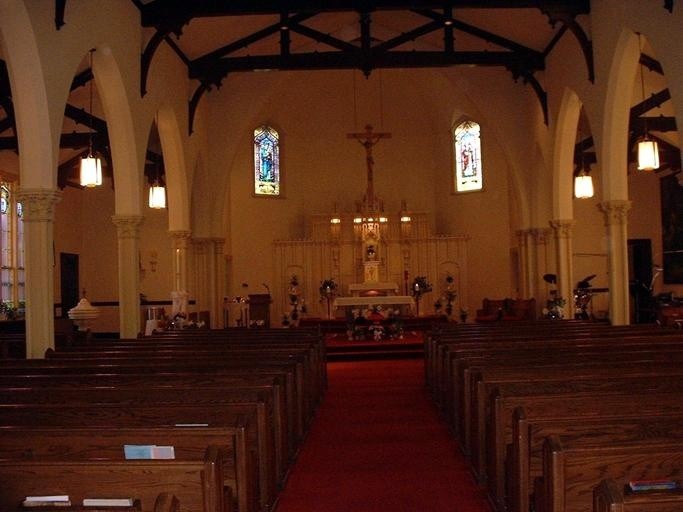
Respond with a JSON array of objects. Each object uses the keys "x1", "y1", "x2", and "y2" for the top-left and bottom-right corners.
[
  {"x1": 459, "y1": 138, "x2": 476, "y2": 178},
  {"x1": 259, "y1": 138, "x2": 274, "y2": 183}
]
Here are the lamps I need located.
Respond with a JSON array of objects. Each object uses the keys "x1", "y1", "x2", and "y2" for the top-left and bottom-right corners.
[
  {"x1": 149, "y1": 119, "x2": 166, "y2": 210},
  {"x1": 574, "y1": 98, "x2": 594, "y2": 199},
  {"x1": 80, "y1": 49, "x2": 102, "y2": 189},
  {"x1": 637, "y1": 32, "x2": 661, "y2": 172}
]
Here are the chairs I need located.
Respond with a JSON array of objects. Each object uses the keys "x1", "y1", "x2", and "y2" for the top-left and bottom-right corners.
[
  {"x1": 502, "y1": 297, "x2": 536, "y2": 322},
  {"x1": 476, "y1": 297, "x2": 505, "y2": 322}
]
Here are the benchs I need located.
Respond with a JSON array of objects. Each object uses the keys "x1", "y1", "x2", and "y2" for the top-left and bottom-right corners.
[
  {"x1": 0, "y1": 328, "x2": 328, "y2": 512},
  {"x1": 421, "y1": 319, "x2": 682, "y2": 512}
]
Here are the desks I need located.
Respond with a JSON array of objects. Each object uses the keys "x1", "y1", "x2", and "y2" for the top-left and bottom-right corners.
[
  {"x1": 332, "y1": 296, "x2": 414, "y2": 321},
  {"x1": 348, "y1": 282, "x2": 400, "y2": 297}
]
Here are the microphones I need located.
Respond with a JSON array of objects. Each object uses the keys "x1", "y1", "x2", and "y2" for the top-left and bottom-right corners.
[{"x1": 262, "y1": 283, "x2": 270, "y2": 293}]
